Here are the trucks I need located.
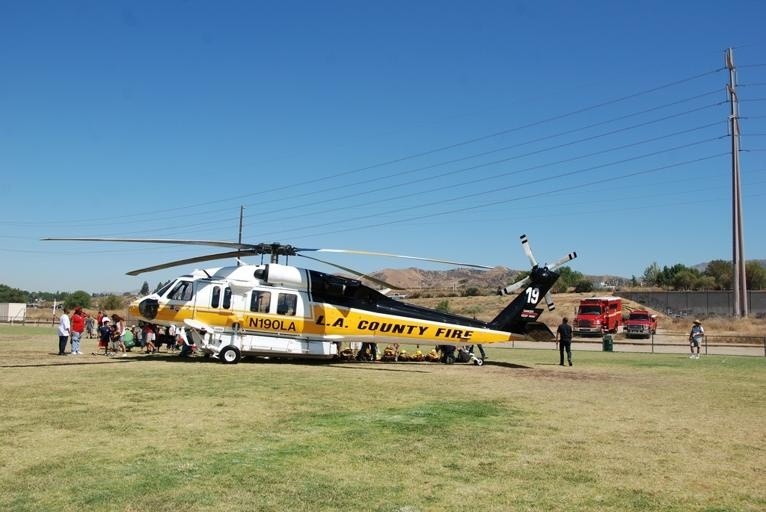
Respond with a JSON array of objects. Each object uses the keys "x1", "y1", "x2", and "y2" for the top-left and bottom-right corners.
[{"x1": 622, "y1": 310, "x2": 657, "y2": 340}]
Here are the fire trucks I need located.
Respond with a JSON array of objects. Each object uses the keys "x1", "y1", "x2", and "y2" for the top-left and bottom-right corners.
[{"x1": 571, "y1": 294, "x2": 623, "y2": 337}]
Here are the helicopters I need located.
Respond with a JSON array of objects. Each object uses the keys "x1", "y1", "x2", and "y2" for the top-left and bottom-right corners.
[{"x1": 36, "y1": 231, "x2": 577, "y2": 369}]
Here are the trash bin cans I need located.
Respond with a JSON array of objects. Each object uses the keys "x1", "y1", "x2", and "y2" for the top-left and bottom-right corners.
[{"x1": 602, "y1": 334, "x2": 613, "y2": 352}]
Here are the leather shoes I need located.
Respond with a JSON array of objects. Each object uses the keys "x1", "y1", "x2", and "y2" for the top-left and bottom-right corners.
[{"x1": 568, "y1": 358, "x2": 572, "y2": 366}]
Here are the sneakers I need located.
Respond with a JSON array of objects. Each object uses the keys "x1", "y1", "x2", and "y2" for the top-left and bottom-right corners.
[
  {"x1": 689, "y1": 353, "x2": 700, "y2": 360},
  {"x1": 58, "y1": 350, "x2": 83, "y2": 355}
]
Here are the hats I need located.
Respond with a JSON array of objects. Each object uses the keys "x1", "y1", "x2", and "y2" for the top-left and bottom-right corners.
[{"x1": 693, "y1": 319, "x2": 702, "y2": 325}]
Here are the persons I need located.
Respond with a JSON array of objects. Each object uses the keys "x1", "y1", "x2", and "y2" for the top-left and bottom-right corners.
[
  {"x1": 56, "y1": 305, "x2": 200, "y2": 359},
  {"x1": 332, "y1": 341, "x2": 378, "y2": 362},
  {"x1": 687, "y1": 319, "x2": 705, "y2": 359},
  {"x1": 556, "y1": 316, "x2": 574, "y2": 367}
]
